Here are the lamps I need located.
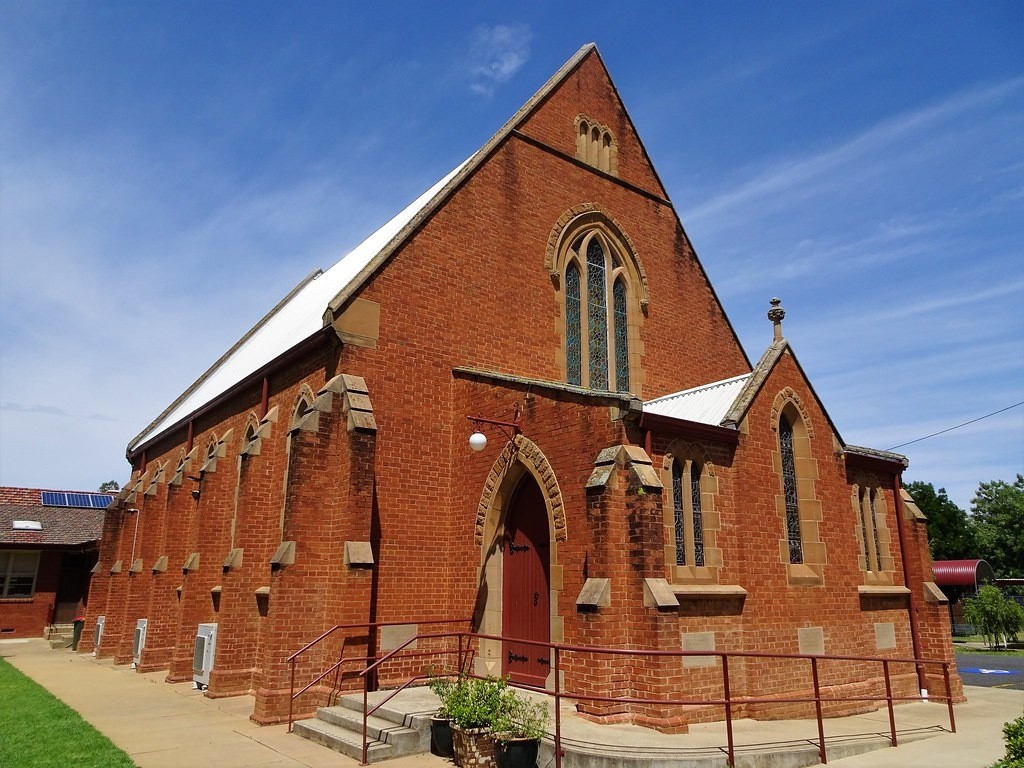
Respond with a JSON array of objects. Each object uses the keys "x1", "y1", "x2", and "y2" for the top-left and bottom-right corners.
[{"x1": 467, "y1": 411, "x2": 522, "y2": 453}]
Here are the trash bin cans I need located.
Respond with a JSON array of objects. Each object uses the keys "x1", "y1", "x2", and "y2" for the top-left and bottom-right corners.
[{"x1": 72, "y1": 619, "x2": 85, "y2": 652}]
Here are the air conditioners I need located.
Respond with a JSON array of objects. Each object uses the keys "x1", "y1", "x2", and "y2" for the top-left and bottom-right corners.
[
  {"x1": 131, "y1": 618, "x2": 147, "y2": 669},
  {"x1": 192, "y1": 623, "x2": 219, "y2": 692},
  {"x1": 93, "y1": 615, "x2": 105, "y2": 657}
]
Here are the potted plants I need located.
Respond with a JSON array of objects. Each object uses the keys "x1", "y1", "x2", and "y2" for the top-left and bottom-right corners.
[{"x1": 422, "y1": 661, "x2": 553, "y2": 768}]
